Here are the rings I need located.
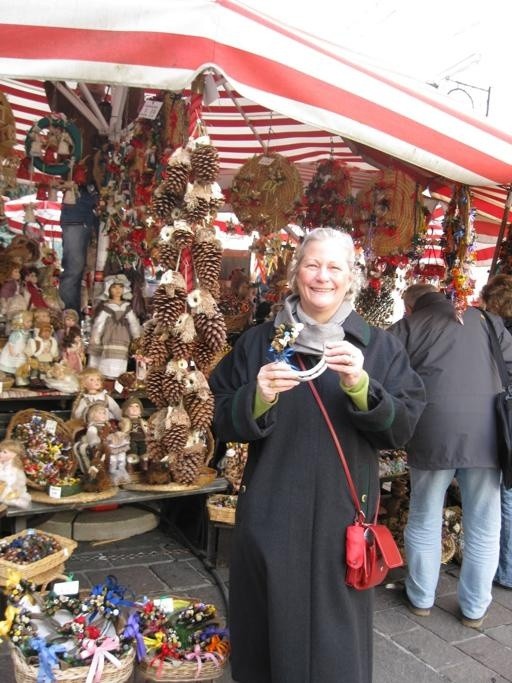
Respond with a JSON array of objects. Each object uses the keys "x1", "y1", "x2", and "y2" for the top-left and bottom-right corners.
[{"x1": 345, "y1": 354, "x2": 355, "y2": 367}]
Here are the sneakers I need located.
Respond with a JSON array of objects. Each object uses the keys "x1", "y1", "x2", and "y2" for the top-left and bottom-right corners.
[
  {"x1": 401, "y1": 587, "x2": 431, "y2": 617},
  {"x1": 460, "y1": 607, "x2": 489, "y2": 629}
]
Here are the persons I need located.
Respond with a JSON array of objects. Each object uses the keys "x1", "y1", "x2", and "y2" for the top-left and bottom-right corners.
[
  {"x1": 68, "y1": 365, "x2": 149, "y2": 489},
  {"x1": 198, "y1": 223, "x2": 432, "y2": 682},
  {"x1": 380, "y1": 282, "x2": 512, "y2": 631},
  {"x1": 89, "y1": 273, "x2": 141, "y2": 381},
  {"x1": 0, "y1": 440, "x2": 34, "y2": 511},
  {"x1": 1, "y1": 77, "x2": 227, "y2": 498},
  {"x1": 0, "y1": 235, "x2": 88, "y2": 390},
  {"x1": 44, "y1": 79, "x2": 114, "y2": 317},
  {"x1": 473, "y1": 269, "x2": 511, "y2": 593}
]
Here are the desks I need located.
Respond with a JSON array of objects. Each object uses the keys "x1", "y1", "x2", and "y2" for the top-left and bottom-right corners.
[{"x1": 1, "y1": 386, "x2": 230, "y2": 621}]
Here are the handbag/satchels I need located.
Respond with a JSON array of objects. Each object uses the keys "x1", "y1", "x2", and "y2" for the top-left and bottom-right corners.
[
  {"x1": 344, "y1": 522, "x2": 403, "y2": 592},
  {"x1": 493, "y1": 389, "x2": 512, "y2": 491}
]
